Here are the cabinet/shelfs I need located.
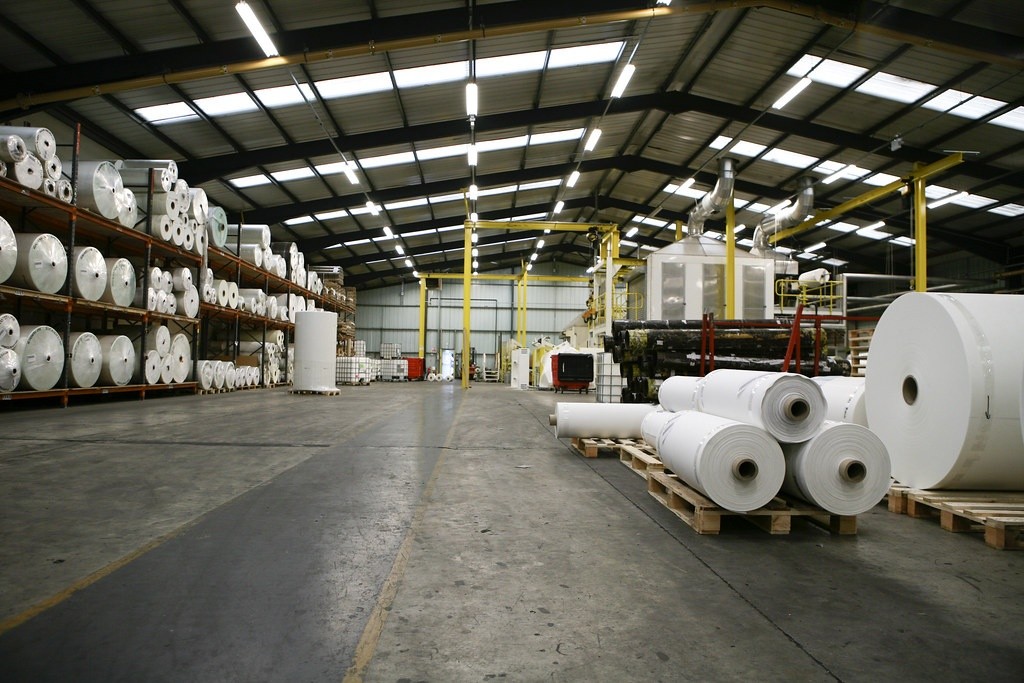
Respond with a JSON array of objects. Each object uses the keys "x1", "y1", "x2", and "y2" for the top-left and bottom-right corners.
[
  {"x1": 196, "y1": 210, "x2": 357, "y2": 395},
  {"x1": 0, "y1": 121, "x2": 206, "y2": 408}
]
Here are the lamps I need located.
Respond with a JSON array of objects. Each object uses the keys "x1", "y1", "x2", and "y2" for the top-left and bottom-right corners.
[{"x1": 235, "y1": 0, "x2": 280, "y2": 57}]
